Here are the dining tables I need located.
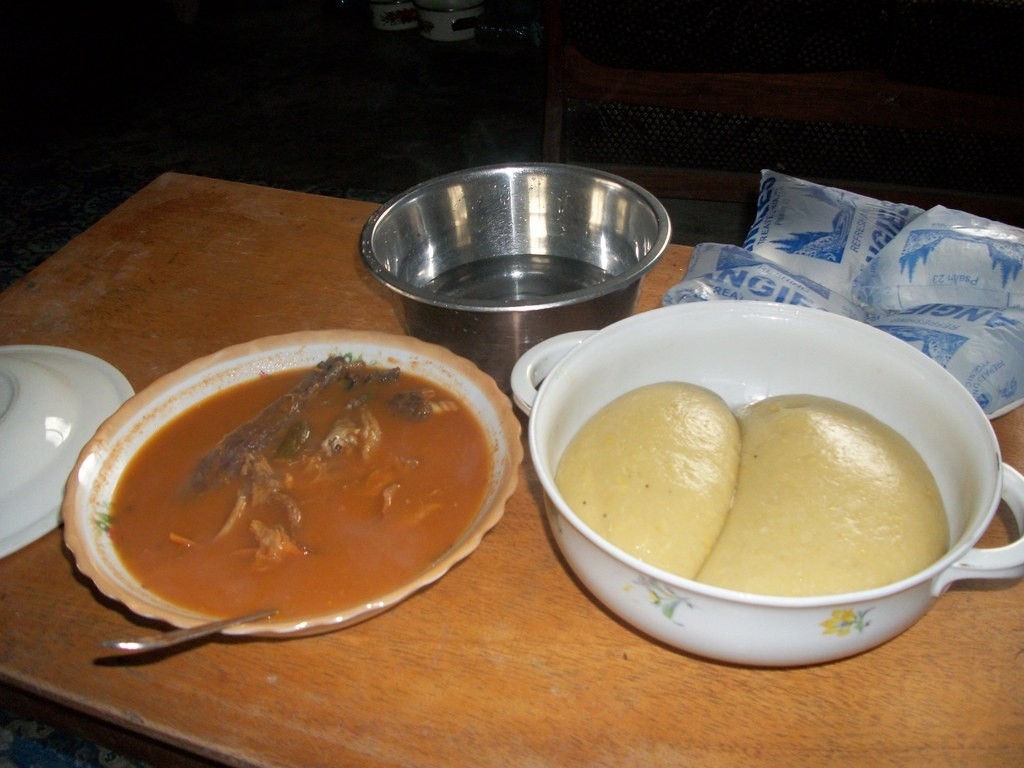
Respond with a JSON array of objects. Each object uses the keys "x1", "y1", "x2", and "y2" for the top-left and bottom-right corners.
[{"x1": 1, "y1": 172, "x2": 1023, "y2": 768}]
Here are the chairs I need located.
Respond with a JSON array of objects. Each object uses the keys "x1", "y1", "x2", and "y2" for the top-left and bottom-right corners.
[{"x1": 545, "y1": 0, "x2": 1023, "y2": 228}]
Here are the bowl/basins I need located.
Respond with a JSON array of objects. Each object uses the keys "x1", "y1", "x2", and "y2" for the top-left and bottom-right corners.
[
  {"x1": 361, "y1": 161, "x2": 672, "y2": 400},
  {"x1": 509, "y1": 300, "x2": 1024, "y2": 668}
]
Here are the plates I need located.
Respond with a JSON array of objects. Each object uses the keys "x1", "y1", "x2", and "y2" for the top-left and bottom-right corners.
[
  {"x1": 61, "y1": 331, "x2": 524, "y2": 640},
  {"x1": 0, "y1": 344, "x2": 136, "y2": 560}
]
